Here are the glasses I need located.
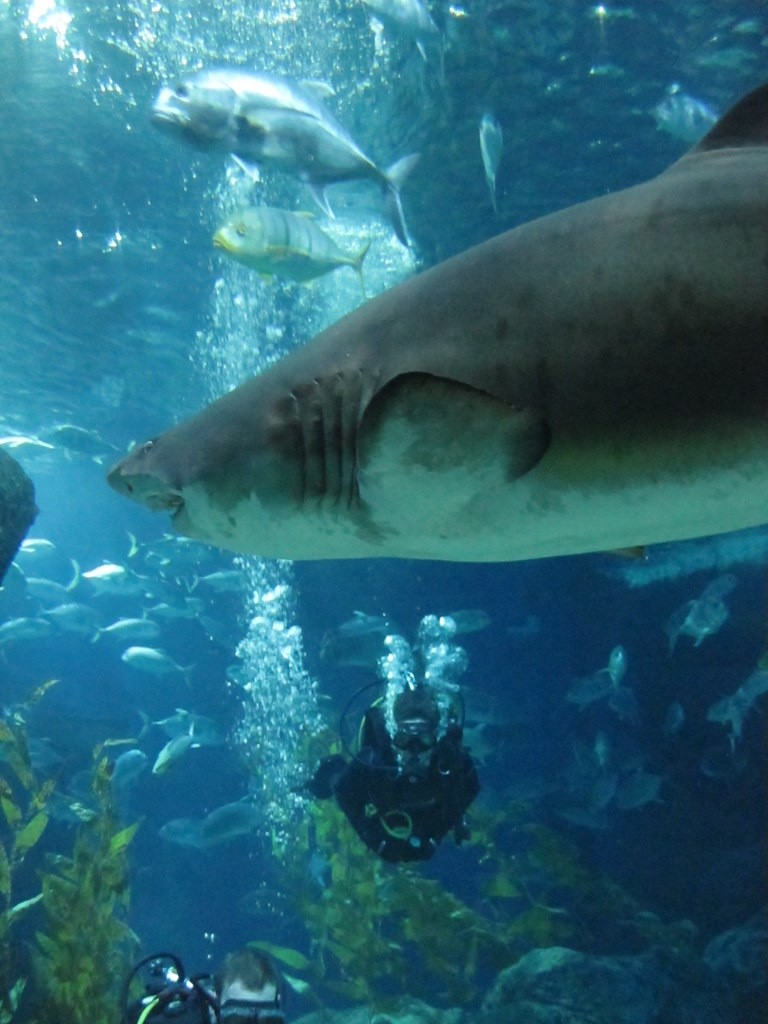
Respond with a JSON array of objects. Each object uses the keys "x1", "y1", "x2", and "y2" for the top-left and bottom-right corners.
[
  {"x1": 219, "y1": 1005, "x2": 285, "y2": 1024},
  {"x1": 392, "y1": 720, "x2": 437, "y2": 750}
]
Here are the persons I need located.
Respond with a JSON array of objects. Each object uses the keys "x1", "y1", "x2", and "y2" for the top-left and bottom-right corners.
[
  {"x1": 122, "y1": 946, "x2": 286, "y2": 1024},
  {"x1": 303, "y1": 680, "x2": 482, "y2": 863}
]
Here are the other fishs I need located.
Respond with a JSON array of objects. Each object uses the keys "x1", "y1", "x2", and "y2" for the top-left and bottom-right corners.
[{"x1": 0, "y1": 0, "x2": 768, "y2": 1024}]
